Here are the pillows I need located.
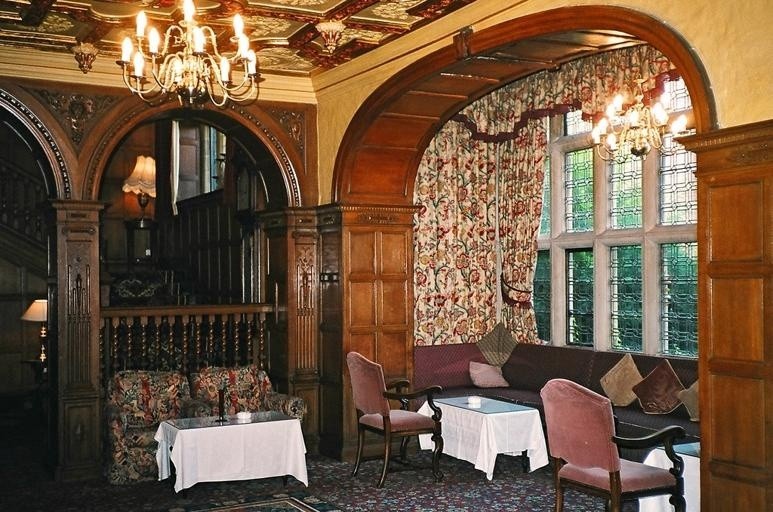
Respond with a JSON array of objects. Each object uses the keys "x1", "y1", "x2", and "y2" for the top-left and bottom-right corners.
[
  {"x1": 468, "y1": 323, "x2": 519, "y2": 391},
  {"x1": 597, "y1": 351, "x2": 701, "y2": 426}
]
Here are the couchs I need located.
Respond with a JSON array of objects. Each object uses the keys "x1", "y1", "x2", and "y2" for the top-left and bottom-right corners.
[{"x1": 99, "y1": 361, "x2": 307, "y2": 482}]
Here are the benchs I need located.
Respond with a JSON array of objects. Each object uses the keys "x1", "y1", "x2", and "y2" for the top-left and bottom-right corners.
[{"x1": 413, "y1": 337, "x2": 700, "y2": 458}]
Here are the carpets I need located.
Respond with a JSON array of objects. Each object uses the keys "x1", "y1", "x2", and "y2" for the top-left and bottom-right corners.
[{"x1": 153, "y1": 491, "x2": 343, "y2": 512}]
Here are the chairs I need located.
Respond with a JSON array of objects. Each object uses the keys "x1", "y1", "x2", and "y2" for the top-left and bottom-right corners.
[
  {"x1": 340, "y1": 349, "x2": 446, "y2": 490},
  {"x1": 538, "y1": 377, "x2": 689, "y2": 512}
]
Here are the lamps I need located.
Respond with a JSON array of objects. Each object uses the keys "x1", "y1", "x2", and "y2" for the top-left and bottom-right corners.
[
  {"x1": 18, "y1": 296, "x2": 54, "y2": 366},
  {"x1": 114, "y1": 1, "x2": 262, "y2": 115},
  {"x1": 583, "y1": 45, "x2": 694, "y2": 168},
  {"x1": 122, "y1": 152, "x2": 160, "y2": 234}
]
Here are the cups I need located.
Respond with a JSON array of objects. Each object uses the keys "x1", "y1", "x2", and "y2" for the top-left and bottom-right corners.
[
  {"x1": 237, "y1": 412, "x2": 251, "y2": 419},
  {"x1": 468, "y1": 396, "x2": 482, "y2": 406}
]
